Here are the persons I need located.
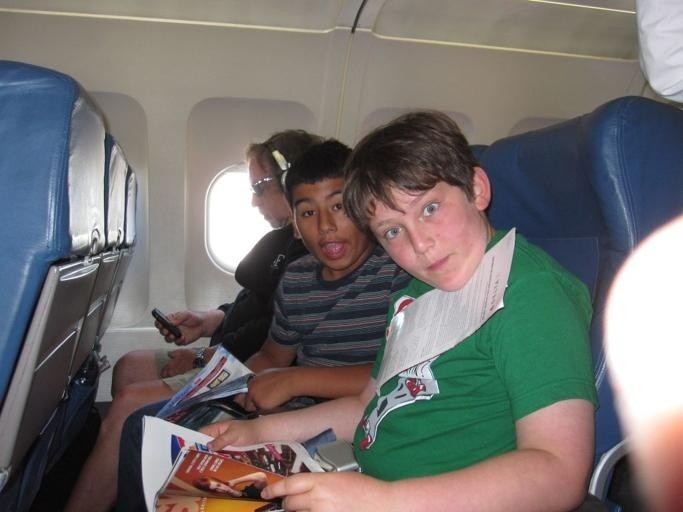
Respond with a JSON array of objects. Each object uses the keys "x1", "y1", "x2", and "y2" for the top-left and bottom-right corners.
[
  {"x1": 197, "y1": 108, "x2": 596, "y2": 512},
  {"x1": 55, "y1": 127, "x2": 329, "y2": 512},
  {"x1": 116, "y1": 139, "x2": 413, "y2": 512}
]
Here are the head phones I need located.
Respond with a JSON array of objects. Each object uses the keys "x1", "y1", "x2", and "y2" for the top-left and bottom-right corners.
[{"x1": 264, "y1": 136, "x2": 293, "y2": 190}]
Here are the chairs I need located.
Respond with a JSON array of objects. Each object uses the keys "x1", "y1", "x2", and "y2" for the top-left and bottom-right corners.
[
  {"x1": 0, "y1": 61, "x2": 138, "y2": 512},
  {"x1": 469, "y1": 95, "x2": 683, "y2": 511}
]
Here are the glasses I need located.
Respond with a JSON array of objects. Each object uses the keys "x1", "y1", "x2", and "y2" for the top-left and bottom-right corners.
[{"x1": 251, "y1": 177, "x2": 276, "y2": 196}]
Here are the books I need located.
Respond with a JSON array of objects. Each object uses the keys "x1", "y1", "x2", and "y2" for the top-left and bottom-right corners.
[
  {"x1": 134, "y1": 407, "x2": 324, "y2": 512},
  {"x1": 154, "y1": 344, "x2": 315, "y2": 435}
]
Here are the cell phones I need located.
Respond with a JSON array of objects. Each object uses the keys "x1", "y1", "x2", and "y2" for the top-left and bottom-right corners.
[{"x1": 151, "y1": 307, "x2": 182, "y2": 340}]
